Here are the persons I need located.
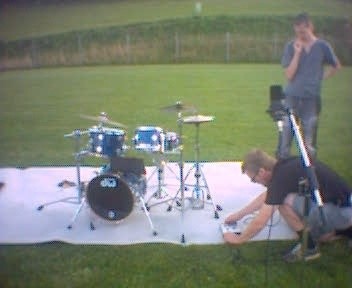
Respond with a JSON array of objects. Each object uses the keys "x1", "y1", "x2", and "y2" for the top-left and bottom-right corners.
[
  {"x1": 273, "y1": 12, "x2": 340, "y2": 161},
  {"x1": 223, "y1": 149, "x2": 352, "y2": 264}
]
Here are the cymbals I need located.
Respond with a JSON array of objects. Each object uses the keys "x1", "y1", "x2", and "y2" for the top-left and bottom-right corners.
[
  {"x1": 159, "y1": 102, "x2": 192, "y2": 112},
  {"x1": 79, "y1": 114, "x2": 128, "y2": 129},
  {"x1": 179, "y1": 115, "x2": 215, "y2": 123}
]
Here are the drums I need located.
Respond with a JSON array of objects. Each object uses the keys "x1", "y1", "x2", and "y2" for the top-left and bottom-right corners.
[
  {"x1": 87, "y1": 131, "x2": 126, "y2": 157},
  {"x1": 161, "y1": 132, "x2": 183, "y2": 154},
  {"x1": 85, "y1": 163, "x2": 147, "y2": 224},
  {"x1": 132, "y1": 127, "x2": 163, "y2": 153}
]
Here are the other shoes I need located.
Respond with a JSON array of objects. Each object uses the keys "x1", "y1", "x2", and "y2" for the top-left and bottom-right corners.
[
  {"x1": 284, "y1": 240, "x2": 321, "y2": 263},
  {"x1": 320, "y1": 225, "x2": 352, "y2": 242}
]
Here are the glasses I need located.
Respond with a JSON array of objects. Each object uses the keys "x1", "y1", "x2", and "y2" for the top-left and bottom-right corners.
[{"x1": 251, "y1": 170, "x2": 260, "y2": 183}]
